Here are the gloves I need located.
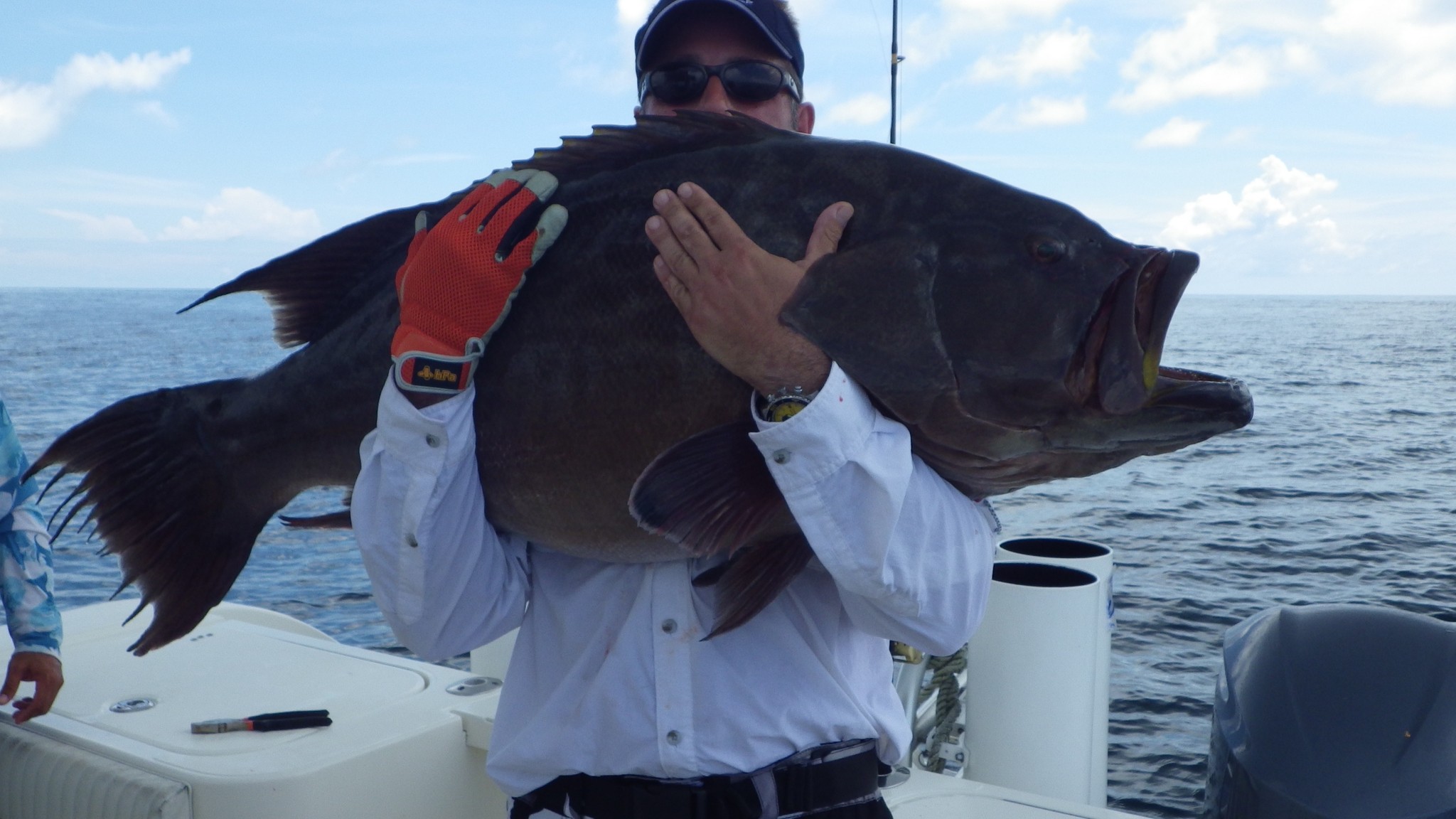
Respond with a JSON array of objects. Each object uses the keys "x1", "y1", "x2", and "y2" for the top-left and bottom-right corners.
[{"x1": 388, "y1": 166, "x2": 574, "y2": 393}]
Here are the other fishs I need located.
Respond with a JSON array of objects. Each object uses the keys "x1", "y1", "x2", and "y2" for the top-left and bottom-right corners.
[{"x1": 20, "y1": 110, "x2": 1253, "y2": 656}]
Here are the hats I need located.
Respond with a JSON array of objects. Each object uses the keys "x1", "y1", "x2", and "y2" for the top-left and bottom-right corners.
[{"x1": 633, "y1": 0, "x2": 804, "y2": 78}]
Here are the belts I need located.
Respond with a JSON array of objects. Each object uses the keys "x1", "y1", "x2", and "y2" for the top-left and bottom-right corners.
[{"x1": 513, "y1": 752, "x2": 885, "y2": 818}]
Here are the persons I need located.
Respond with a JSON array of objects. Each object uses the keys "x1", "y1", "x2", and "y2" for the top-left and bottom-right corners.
[
  {"x1": 341, "y1": 2, "x2": 1005, "y2": 817},
  {"x1": 0, "y1": 395, "x2": 69, "y2": 731}
]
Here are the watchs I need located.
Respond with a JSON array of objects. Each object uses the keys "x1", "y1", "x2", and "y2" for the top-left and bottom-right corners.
[{"x1": 757, "y1": 386, "x2": 818, "y2": 425}]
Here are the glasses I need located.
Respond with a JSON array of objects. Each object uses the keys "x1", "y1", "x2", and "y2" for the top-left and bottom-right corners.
[{"x1": 640, "y1": 58, "x2": 801, "y2": 102}]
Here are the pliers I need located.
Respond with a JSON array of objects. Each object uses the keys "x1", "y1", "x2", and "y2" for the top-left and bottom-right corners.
[{"x1": 190, "y1": 709, "x2": 332, "y2": 734}]
